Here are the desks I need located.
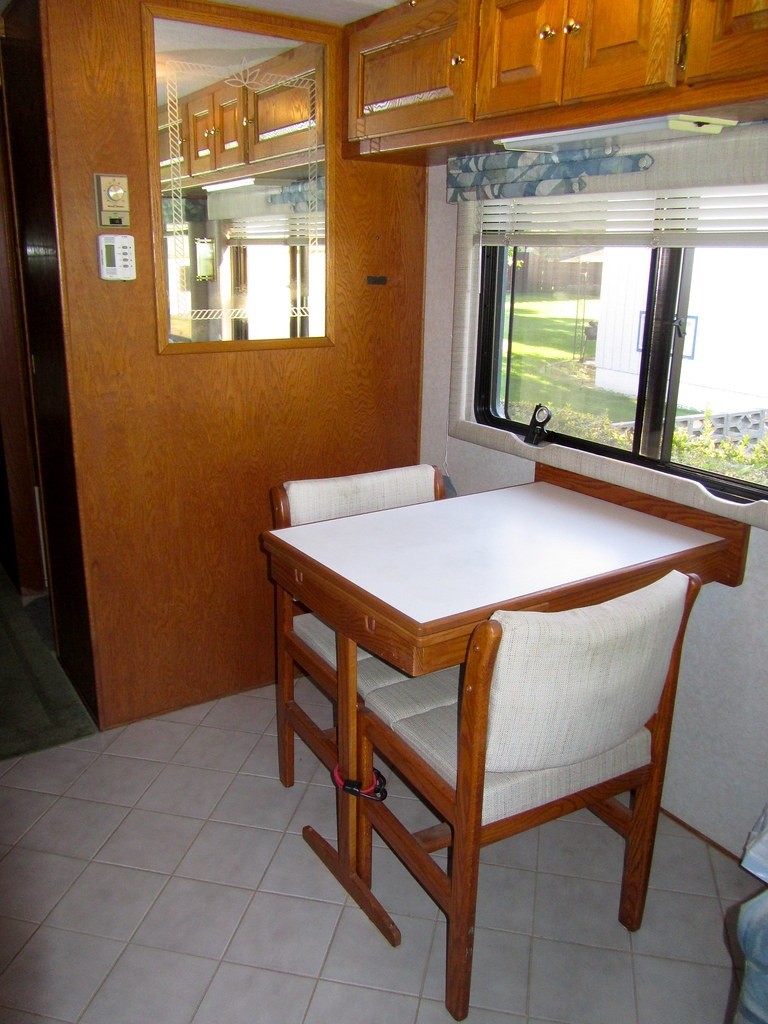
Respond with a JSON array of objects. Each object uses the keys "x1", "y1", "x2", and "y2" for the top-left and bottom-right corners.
[{"x1": 258, "y1": 462, "x2": 751, "y2": 950}]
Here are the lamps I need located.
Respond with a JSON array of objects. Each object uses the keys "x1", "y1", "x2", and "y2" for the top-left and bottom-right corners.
[
  {"x1": 195, "y1": 237, "x2": 217, "y2": 282},
  {"x1": 490, "y1": 112, "x2": 737, "y2": 155},
  {"x1": 201, "y1": 177, "x2": 297, "y2": 195}
]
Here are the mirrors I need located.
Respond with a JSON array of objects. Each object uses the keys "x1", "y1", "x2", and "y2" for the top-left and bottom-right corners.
[{"x1": 137, "y1": 1, "x2": 336, "y2": 358}]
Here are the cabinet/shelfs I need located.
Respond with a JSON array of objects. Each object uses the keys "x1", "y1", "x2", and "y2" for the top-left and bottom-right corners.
[
  {"x1": 243, "y1": 40, "x2": 326, "y2": 181},
  {"x1": 478, "y1": 0, "x2": 716, "y2": 158},
  {"x1": 156, "y1": 97, "x2": 203, "y2": 199},
  {"x1": 187, "y1": 70, "x2": 266, "y2": 192},
  {"x1": 343, "y1": 0, "x2": 477, "y2": 156},
  {"x1": 683, "y1": 2, "x2": 768, "y2": 127}
]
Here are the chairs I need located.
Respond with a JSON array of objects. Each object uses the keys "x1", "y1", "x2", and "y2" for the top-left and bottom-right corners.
[
  {"x1": 359, "y1": 569, "x2": 700, "y2": 1023},
  {"x1": 268, "y1": 464, "x2": 445, "y2": 787}
]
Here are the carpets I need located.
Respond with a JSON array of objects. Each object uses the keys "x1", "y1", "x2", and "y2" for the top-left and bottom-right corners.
[{"x1": 0, "y1": 580, "x2": 99, "y2": 763}]
